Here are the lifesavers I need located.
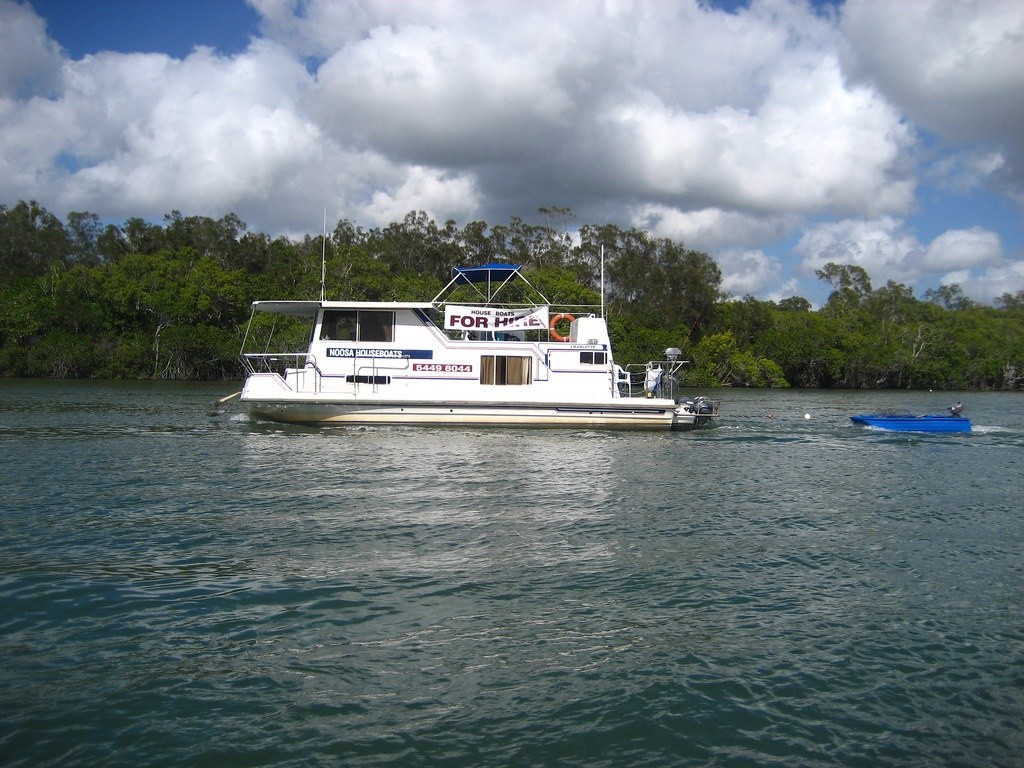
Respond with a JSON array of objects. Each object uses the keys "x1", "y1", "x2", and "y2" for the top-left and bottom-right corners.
[{"x1": 549, "y1": 314, "x2": 576, "y2": 342}]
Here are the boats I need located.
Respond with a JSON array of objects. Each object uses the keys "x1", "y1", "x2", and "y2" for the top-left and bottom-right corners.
[
  {"x1": 849, "y1": 401, "x2": 975, "y2": 432},
  {"x1": 206, "y1": 204, "x2": 721, "y2": 431}
]
